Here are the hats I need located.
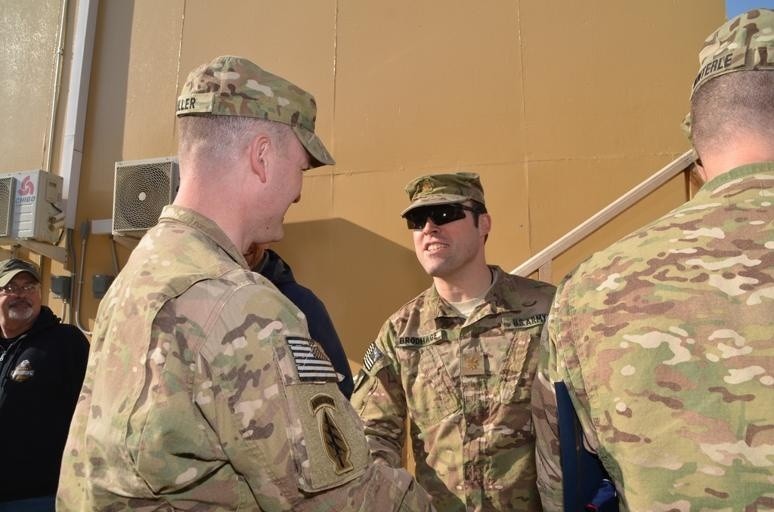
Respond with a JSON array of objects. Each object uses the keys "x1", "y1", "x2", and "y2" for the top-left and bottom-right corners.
[
  {"x1": 400, "y1": 172, "x2": 486, "y2": 222},
  {"x1": 690, "y1": 7, "x2": 773, "y2": 98},
  {"x1": 0, "y1": 259, "x2": 41, "y2": 288},
  {"x1": 176, "y1": 56, "x2": 337, "y2": 166}
]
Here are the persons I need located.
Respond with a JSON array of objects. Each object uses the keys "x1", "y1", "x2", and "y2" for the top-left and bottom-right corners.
[
  {"x1": 530, "y1": 6, "x2": 773, "y2": 510},
  {"x1": 240, "y1": 238, "x2": 355, "y2": 404},
  {"x1": 352, "y1": 171, "x2": 559, "y2": 510},
  {"x1": 0, "y1": 255, "x2": 88, "y2": 511},
  {"x1": 54, "y1": 56, "x2": 438, "y2": 510}
]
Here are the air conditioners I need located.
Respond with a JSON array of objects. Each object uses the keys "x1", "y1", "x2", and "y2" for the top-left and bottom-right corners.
[
  {"x1": 2, "y1": 170, "x2": 63, "y2": 242},
  {"x1": 105, "y1": 158, "x2": 183, "y2": 239}
]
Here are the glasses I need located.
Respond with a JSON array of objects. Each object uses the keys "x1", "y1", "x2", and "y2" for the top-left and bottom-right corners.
[
  {"x1": 6, "y1": 284, "x2": 41, "y2": 293},
  {"x1": 406, "y1": 204, "x2": 476, "y2": 229}
]
